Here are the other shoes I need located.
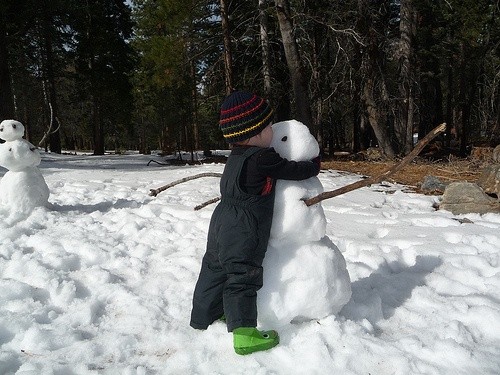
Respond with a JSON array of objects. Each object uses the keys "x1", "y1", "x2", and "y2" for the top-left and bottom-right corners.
[
  {"x1": 232, "y1": 326, "x2": 280, "y2": 355},
  {"x1": 220, "y1": 314, "x2": 226, "y2": 321}
]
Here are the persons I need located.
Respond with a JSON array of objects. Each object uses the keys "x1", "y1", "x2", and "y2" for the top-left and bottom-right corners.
[{"x1": 190, "y1": 92, "x2": 320, "y2": 355}]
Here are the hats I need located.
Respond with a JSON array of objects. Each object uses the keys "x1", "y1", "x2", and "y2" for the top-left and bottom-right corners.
[{"x1": 218, "y1": 90, "x2": 274, "y2": 143}]
